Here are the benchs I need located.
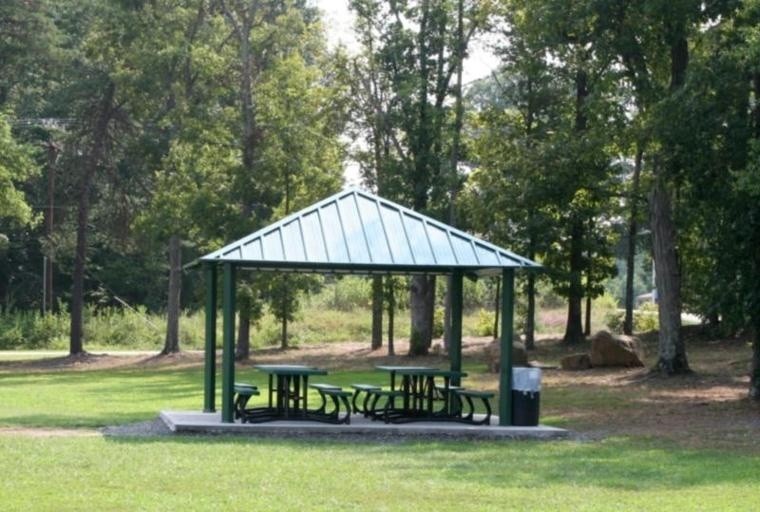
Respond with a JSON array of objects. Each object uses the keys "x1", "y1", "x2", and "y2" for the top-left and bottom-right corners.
[{"x1": 231, "y1": 362, "x2": 493, "y2": 428}]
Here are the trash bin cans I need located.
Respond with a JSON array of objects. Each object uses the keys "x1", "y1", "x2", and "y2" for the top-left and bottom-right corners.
[{"x1": 511, "y1": 367, "x2": 542, "y2": 426}]
